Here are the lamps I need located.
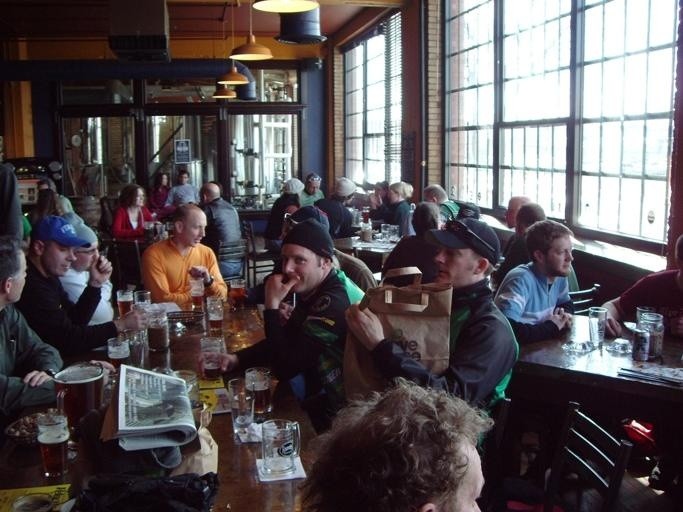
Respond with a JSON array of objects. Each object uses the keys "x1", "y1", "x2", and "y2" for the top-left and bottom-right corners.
[{"x1": 211, "y1": 0, "x2": 319, "y2": 99}]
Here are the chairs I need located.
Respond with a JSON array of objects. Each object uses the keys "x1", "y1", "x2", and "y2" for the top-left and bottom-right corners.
[
  {"x1": 352, "y1": 246, "x2": 383, "y2": 274},
  {"x1": 542, "y1": 400, "x2": 673, "y2": 511},
  {"x1": 216, "y1": 219, "x2": 274, "y2": 288},
  {"x1": 568, "y1": 282, "x2": 601, "y2": 315}
]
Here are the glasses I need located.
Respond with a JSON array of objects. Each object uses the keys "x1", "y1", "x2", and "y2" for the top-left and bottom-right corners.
[{"x1": 446, "y1": 220, "x2": 494, "y2": 251}]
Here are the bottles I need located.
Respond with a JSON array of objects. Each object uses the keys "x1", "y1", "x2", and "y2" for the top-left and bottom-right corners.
[
  {"x1": 362, "y1": 206, "x2": 371, "y2": 223},
  {"x1": 360, "y1": 224, "x2": 372, "y2": 241},
  {"x1": 636, "y1": 305, "x2": 665, "y2": 360}
]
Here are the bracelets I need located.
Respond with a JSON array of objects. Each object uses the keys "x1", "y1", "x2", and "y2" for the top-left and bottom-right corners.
[
  {"x1": 204, "y1": 277, "x2": 214, "y2": 287},
  {"x1": 45, "y1": 369, "x2": 55, "y2": 376}
]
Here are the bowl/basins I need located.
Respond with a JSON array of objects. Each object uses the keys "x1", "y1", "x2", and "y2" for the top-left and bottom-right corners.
[
  {"x1": 3, "y1": 414, "x2": 35, "y2": 450},
  {"x1": 606, "y1": 337, "x2": 633, "y2": 355}
]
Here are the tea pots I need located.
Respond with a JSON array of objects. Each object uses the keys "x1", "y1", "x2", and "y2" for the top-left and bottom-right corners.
[{"x1": 55, "y1": 361, "x2": 103, "y2": 414}]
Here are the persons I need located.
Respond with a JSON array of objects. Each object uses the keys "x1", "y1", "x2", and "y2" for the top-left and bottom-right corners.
[
  {"x1": 492, "y1": 220, "x2": 576, "y2": 347},
  {"x1": 490, "y1": 202, "x2": 588, "y2": 316},
  {"x1": 597, "y1": 235, "x2": 682, "y2": 489},
  {"x1": 109, "y1": 170, "x2": 242, "y2": 276},
  {"x1": 263, "y1": 171, "x2": 480, "y2": 290},
  {"x1": 2, "y1": 166, "x2": 114, "y2": 350},
  {"x1": 343, "y1": 217, "x2": 520, "y2": 457},
  {"x1": 15, "y1": 216, "x2": 147, "y2": 357},
  {"x1": 142, "y1": 204, "x2": 227, "y2": 303},
  {"x1": 198, "y1": 218, "x2": 366, "y2": 434},
  {"x1": 501, "y1": 195, "x2": 532, "y2": 257},
  {"x1": 298, "y1": 377, "x2": 494, "y2": 512},
  {"x1": 1, "y1": 235, "x2": 63, "y2": 413}
]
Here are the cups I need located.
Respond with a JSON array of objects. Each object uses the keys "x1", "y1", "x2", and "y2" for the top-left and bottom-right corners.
[
  {"x1": 246, "y1": 365, "x2": 270, "y2": 414},
  {"x1": 142, "y1": 219, "x2": 173, "y2": 240},
  {"x1": 189, "y1": 278, "x2": 204, "y2": 310},
  {"x1": 106, "y1": 336, "x2": 129, "y2": 363},
  {"x1": 349, "y1": 209, "x2": 360, "y2": 225},
  {"x1": 231, "y1": 277, "x2": 246, "y2": 305},
  {"x1": 259, "y1": 419, "x2": 305, "y2": 478},
  {"x1": 134, "y1": 289, "x2": 150, "y2": 316},
  {"x1": 126, "y1": 330, "x2": 145, "y2": 368},
  {"x1": 225, "y1": 377, "x2": 256, "y2": 438},
  {"x1": 380, "y1": 223, "x2": 399, "y2": 239},
  {"x1": 117, "y1": 289, "x2": 134, "y2": 325},
  {"x1": 38, "y1": 407, "x2": 70, "y2": 446},
  {"x1": 200, "y1": 337, "x2": 225, "y2": 382},
  {"x1": 410, "y1": 203, "x2": 415, "y2": 210},
  {"x1": 205, "y1": 296, "x2": 225, "y2": 322},
  {"x1": 145, "y1": 312, "x2": 169, "y2": 351},
  {"x1": 176, "y1": 370, "x2": 201, "y2": 414},
  {"x1": 587, "y1": 306, "x2": 607, "y2": 343},
  {"x1": 561, "y1": 340, "x2": 594, "y2": 355},
  {"x1": 11, "y1": 487, "x2": 77, "y2": 511}
]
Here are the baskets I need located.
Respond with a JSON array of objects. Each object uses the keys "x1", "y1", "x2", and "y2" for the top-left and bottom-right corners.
[
  {"x1": 4, "y1": 414, "x2": 41, "y2": 448},
  {"x1": 167, "y1": 311, "x2": 205, "y2": 324}
]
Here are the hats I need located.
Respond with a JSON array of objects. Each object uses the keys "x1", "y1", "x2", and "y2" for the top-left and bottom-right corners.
[
  {"x1": 285, "y1": 177, "x2": 356, "y2": 258},
  {"x1": 425, "y1": 217, "x2": 500, "y2": 265},
  {"x1": 32, "y1": 212, "x2": 98, "y2": 248}
]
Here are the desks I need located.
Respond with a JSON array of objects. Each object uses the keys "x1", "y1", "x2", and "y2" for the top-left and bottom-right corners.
[
  {"x1": 498, "y1": 310, "x2": 683, "y2": 511},
  {"x1": 236, "y1": 209, "x2": 272, "y2": 218}
]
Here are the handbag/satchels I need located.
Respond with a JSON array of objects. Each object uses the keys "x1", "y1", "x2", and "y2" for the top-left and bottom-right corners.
[
  {"x1": 342, "y1": 266, "x2": 454, "y2": 398},
  {"x1": 79, "y1": 472, "x2": 220, "y2": 512}
]
